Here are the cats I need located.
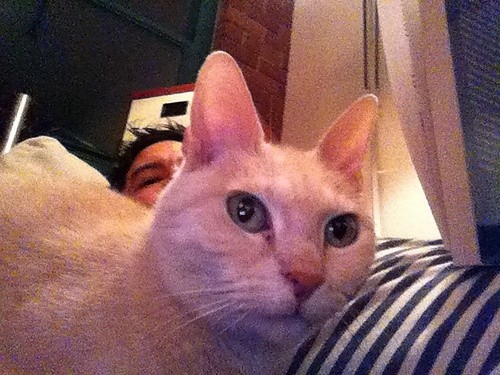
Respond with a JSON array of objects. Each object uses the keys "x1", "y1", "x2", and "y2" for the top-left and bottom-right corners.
[{"x1": 0, "y1": 50, "x2": 379, "y2": 375}]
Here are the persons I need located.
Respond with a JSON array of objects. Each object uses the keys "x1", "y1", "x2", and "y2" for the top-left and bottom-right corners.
[{"x1": 114, "y1": 121, "x2": 500, "y2": 375}]
